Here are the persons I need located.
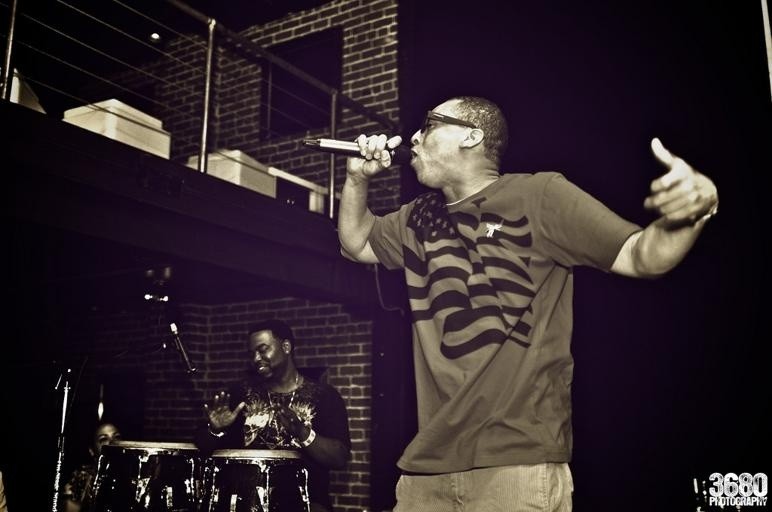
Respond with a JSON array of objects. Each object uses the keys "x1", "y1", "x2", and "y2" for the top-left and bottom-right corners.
[
  {"x1": 63, "y1": 419, "x2": 124, "y2": 512},
  {"x1": 337, "y1": 95, "x2": 718, "y2": 512},
  {"x1": 203, "y1": 319, "x2": 350, "y2": 512}
]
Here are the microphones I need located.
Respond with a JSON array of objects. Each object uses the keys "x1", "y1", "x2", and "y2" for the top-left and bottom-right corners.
[
  {"x1": 302, "y1": 137, "x2": 412, "y2": 166},
  {"x1": 170, "y1": 321, "x2": 201, "y2": 379}
]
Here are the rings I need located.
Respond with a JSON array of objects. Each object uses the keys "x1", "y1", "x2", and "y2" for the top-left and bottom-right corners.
[{"x1": 291, "y1": 418, "x2": 293, "y2": 422}]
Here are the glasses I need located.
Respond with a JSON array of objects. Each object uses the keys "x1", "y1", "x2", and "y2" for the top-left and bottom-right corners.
[{"x1": 421, "y1": 110, "x2": 476, "y2": 133}]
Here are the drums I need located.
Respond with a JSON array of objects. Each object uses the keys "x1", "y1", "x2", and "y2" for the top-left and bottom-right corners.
[
  {"x1": 88, "y1": 439, "x2": 208, "y2": 512},
  {"x1": 201, "y1": 449, "x2": 311, "y2": 512}
]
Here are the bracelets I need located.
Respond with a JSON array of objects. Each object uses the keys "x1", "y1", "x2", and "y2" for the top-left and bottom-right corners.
[
  {"x1": 206, "y1": 424, "x2": 226, "y2": 437},
  {"x1": 298, "y1": 427, "x2": 316, "y2": 448}
]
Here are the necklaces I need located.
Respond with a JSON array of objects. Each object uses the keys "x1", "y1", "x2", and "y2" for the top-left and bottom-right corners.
[{"x1": 266, "y1": 369, "x2": 299, "y2": 442}]
